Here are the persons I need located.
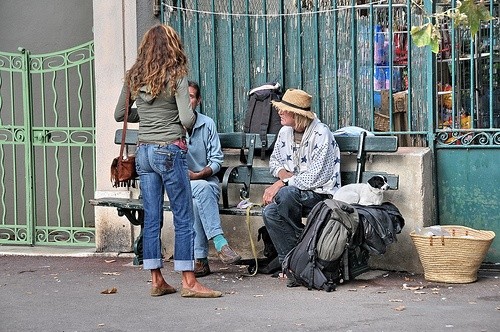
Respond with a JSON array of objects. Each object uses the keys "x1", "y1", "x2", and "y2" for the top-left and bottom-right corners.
[
  {"x1": 262, "y1": 87, "x2": 342, "y2": 278},
  {"x1": 114, "y1": 24, "x2": 222, "y2": 298},
  {"x1": 182, "y1": 80, "x2": 242, "y2": 278}
]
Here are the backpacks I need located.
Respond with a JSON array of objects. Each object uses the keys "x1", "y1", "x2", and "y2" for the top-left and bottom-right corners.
[
  {"x1": 239, "y1": 81, "x2": 283, "y2": 164},
  {"x1": 282, "y1": 199, "x2": 360, "y2": 292}
]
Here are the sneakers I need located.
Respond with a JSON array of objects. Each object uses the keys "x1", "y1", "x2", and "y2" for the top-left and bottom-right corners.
[
  {"x1": 151, "y1": 286, "x2": 177, "y2": 296},
  {"x1": 181, "y1": 288, "x2": 222, "y2": 298}
]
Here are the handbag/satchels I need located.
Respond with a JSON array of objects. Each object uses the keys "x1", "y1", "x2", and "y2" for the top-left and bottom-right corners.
[{"x1": 110, "y1": 153, "x2": 139, "y2": 188}]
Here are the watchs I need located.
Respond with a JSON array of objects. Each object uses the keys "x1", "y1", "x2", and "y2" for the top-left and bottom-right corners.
[{"x1": 282, "y1": 179, "x2": 289, "y2": 186}]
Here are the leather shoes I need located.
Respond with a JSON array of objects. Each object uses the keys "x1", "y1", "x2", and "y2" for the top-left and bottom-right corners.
[
  {"x1": 215, "y1": 242, "x2": 240, "y2": 264},
  {"x1": 194, "y1": 257, "x2": 210, "y2": 277}
]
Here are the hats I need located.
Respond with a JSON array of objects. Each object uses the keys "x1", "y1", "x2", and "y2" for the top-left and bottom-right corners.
[{"x1": 269, "y1": 88, "x2": 315, "y2": 119}]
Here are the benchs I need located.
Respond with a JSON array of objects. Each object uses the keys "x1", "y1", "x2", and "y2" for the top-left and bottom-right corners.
[{"x1": 88, "y1": 132, "x2": 399, "y2": 281}]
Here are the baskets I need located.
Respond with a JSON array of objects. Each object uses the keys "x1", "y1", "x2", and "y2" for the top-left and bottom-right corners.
[
  {"x1": 409, "y1": 224, "x2": 496, "y2": 283},
  {"x1": 374, "y1": 111, "x2": 390, "y2": 131}
]
